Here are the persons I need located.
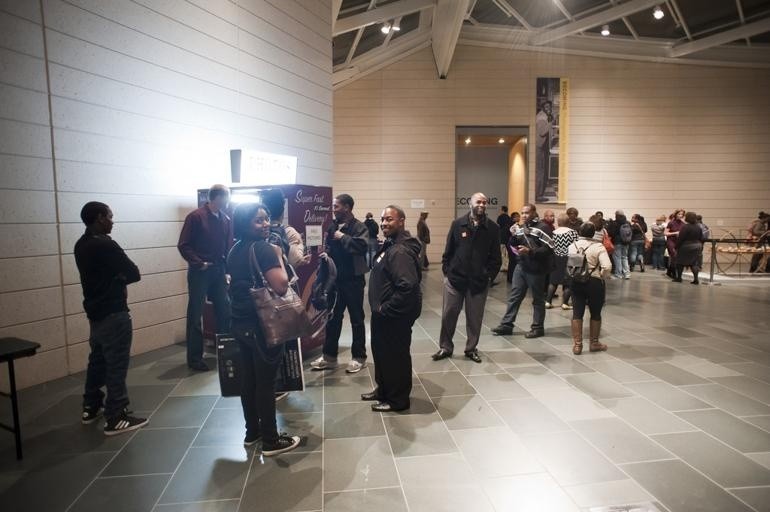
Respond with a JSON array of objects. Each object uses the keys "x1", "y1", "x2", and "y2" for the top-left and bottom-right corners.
[
  {"x1": 567, "y1": 222, "x2": 612, "y2": 355},
  {"x1": 432, "y1": 191, "x2": 503, "y2": 363},
  {"x1": 538, "y1": 210, "x2": 555, "y2": 239},
  {"x1": 497, "y1": 205, "x2": 510, "y2": 228},
  {"x1": 309, "y1": 193, "x2": 369, "y2": 373},
  {"x1": 609, "y1": 210, "x2": 632, "y2": 280},
  {"x1": 663, "y1": 207, "x2": 686, "y2": 281},
  {"x1": 765, "y1": 215, "x2": 770, "y2": 273},
  {"x1": 746, "y1": 212, "x2": 768, "y2": 274},
  {"x1": 417, "y1": 210, "x2": 431, "y2": 270},
  {"x1": 255, "y1": 188, "x2": 312, "y2": 280},
  {"x1": 73, "y1": 201, "x2": 149, "y2": 435},
  {"x1": 364, "y1": 212, "x2": 380, "y2": 271},
  {"x1": 177, "y1": 183, "x2": 233, "y2": 373},
  {"x1": 595, "y1": 210, "x2": 605, "y2": 222},
  {"x1": 566, "y1": 207, "x2": 583, "y2": 230},
  {"x1": 630, "y1": 213, "x2": 646, "y2": 272},
  {"x1": 588, "y1": 215, "x2": 605, "y2": 243},
  {"x1": 491, "y1": 204, "x2": 552, "y2": 338},
  {"x1": 673, "y1": 212, "x2": 701, "y2": 285},
  {"x1": 640, "y1": 217, "x2": 648, "y2": 234},
  {"x1": 545, "y1": 214, "x2": 578, "y2": 310},
  {"x1": 535, "y1": 101, "x2": 553, "y2": 203},
  {"x1": 225, "y1": 202, "x2": 302, "y2": 456},
  {"x1": 361, "y1": 204, "x2": 423, "y2": 411},
  {"x1": 506, "y1": 212, "x2": 521, "y2": 284},
  {"x1": 650, "y1": 214, "x2": 668, "y2": 272},
  {"x1": 666, "y1": 213, "x2": 682, "y2": 278},
  {"x1": 696, "y1": 214, "x2": 711, "y2": 245}
]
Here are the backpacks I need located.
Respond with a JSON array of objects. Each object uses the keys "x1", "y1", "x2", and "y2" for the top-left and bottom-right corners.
[
  {"x1": 747, "y1": 219, "x2": 762, "y2": 240},
  {"x1": 568, "y1": 240, "x2": 603, "y2": 283},
  {"x1": 613, "y1": 221, "x2": 633, "y2": 242},
  {"x1": 266, "y1": 223, "x2": 290, "y2": 254}
]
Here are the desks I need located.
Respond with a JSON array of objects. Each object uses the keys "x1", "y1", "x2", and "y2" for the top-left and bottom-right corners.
[
  {"x1": 653, "y1": 5, "x2": 664, "y2": 19},
  {"x1": 0, "y1": 337, "x2": 42, "y2": 461}
]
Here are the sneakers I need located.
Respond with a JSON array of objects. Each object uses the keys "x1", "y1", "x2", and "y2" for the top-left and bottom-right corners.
[
  {"x1": 544, "y1": 302, "x2": 553, "y2": 309},
  {"x1": 102, "y1": 414, "x2": 149, "y2": 437},
  {"x1": 243, "y1": 434, "x2": 263, "y2": 446},
  {"x1": 561, "y1": 303, "x2": 573, "y2": 310},
  {"x1": 80, "y1": 407, "x2": 104, "y2": 425},
  {"x1": 346, "y1": 359, "x2": 367, "y2": 372},
  {"x1": 262, "y1": 435, "x2": 302, "y2": 457},
  {"x1": 311, "y1": 357, "x2": 338, "y2": 370}
]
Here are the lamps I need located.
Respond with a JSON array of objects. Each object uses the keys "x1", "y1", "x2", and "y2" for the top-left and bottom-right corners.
[
  {"x1": 392, "y1": 16, "x2": 402, "y2": 31},
  {"x1": 381, "y1": 22, "x2": 391, "y2": 34},
  {"x1": 601, "y1": 25, "x2": 610, "y2": 36}
]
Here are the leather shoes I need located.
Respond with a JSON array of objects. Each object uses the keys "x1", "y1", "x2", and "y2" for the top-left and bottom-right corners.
[
  {"x1": 361, "y1": 392, "x2": 378, "y2": 400},
  {"x1": 431, "y1": 349, "x2": 448, "y2": 359},
  {"x1": 525, "y1": 328, "x2": 545, "y2": 338},
  {"x1": 466, "y1": 353, "x2": 482, "y2": 362},
  {"x1": 491, "y1": 325, "x2": 514, "y2": 335},
  {"x1": 371, "y1": 402, "x2": 392, "y2": 412}
]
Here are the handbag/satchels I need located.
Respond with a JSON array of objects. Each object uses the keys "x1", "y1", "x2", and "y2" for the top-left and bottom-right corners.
[
  {"x1": 311, "y1": 254, "x2": 338, "y2": 311},
  {"x1": 636, "y1": 222, "x2": 652, "y2": 253},
  {"x1": 602, "y1": 228, "x2": 615, "y2": 253},
  {"x1": 248, "y1": 241, "x2": 314, "y2": 350}
]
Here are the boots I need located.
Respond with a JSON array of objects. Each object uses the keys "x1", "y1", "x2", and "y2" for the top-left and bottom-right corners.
[
  {"x1": 589, "y1": 319, "x2": 608, "y2": 351},
  {"x1": 571, "y1": 318, "x2": 583, "y2": 355}
]
